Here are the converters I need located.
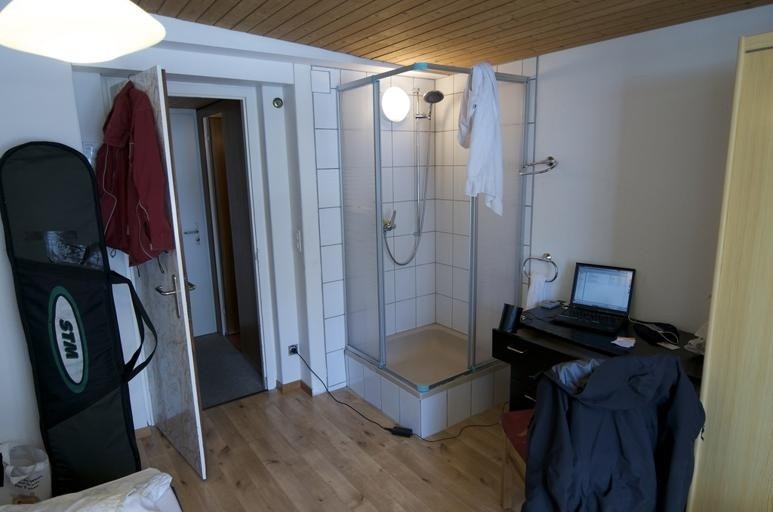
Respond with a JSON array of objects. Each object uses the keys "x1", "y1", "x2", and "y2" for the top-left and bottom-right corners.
[{"x1": 391, "y1": 427, "x2": 412, "y2": 438}]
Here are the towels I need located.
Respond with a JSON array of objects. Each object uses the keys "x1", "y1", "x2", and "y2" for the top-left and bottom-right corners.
[{"x1": 458, "y1": 58, "x2": 505, "y2": 216}]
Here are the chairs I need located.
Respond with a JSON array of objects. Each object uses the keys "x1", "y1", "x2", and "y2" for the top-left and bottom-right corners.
[{"x1": 501, "y1": 357, "x2": 678, "y2": 509}]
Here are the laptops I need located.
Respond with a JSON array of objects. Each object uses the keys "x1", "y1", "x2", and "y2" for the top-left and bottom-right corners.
[{"x1": 552, "y1": 262, "x2": 636, "y2": 334}]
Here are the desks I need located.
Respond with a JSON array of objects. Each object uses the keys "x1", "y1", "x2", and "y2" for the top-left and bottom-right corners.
[{"x1": 493, "y1": 298, "x2": 707, "y2": 412}]
